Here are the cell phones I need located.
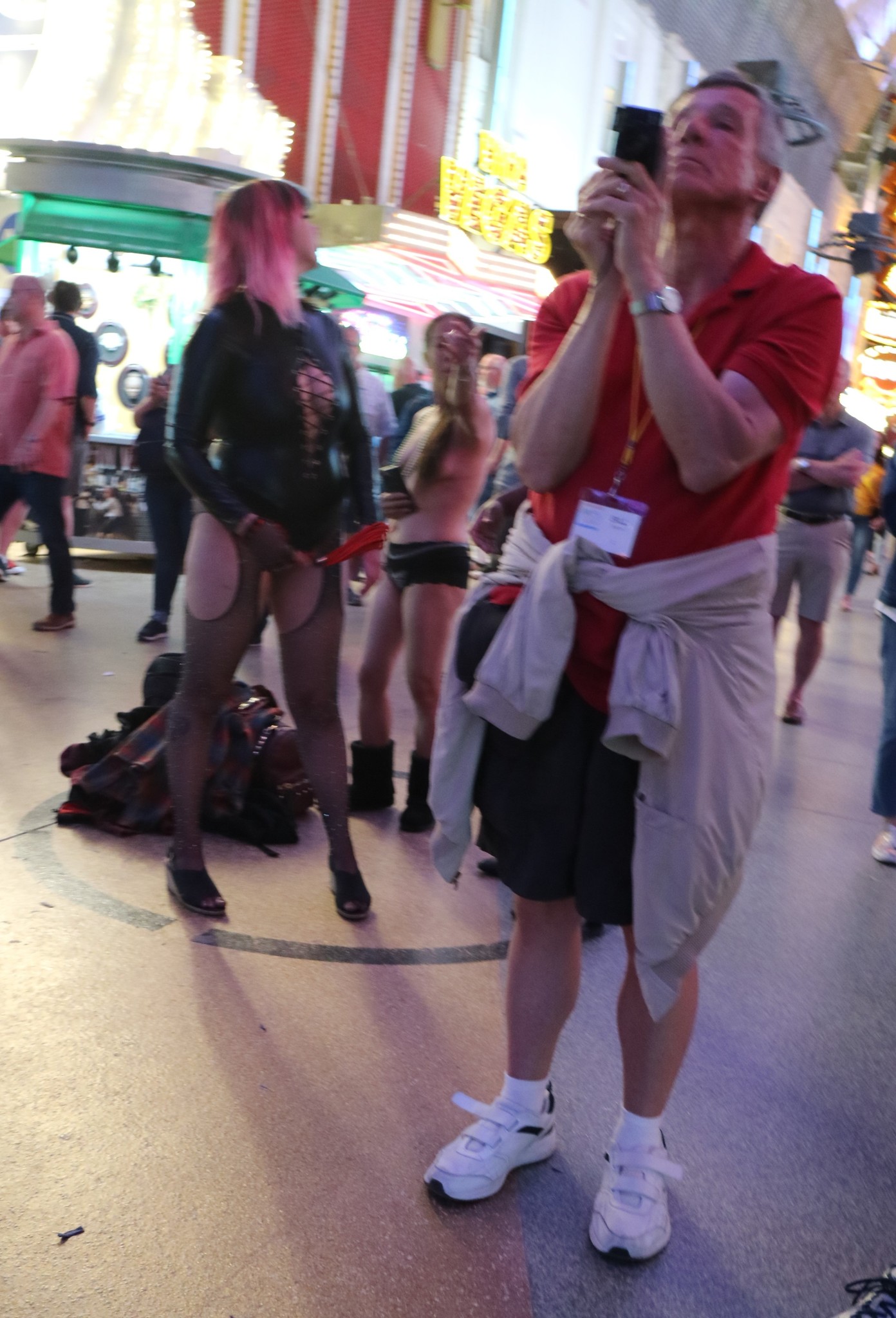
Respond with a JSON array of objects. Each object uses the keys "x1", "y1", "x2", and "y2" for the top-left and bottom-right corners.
[{"x1": 609, "y1": 104, "x2": 665, "y2": 181}]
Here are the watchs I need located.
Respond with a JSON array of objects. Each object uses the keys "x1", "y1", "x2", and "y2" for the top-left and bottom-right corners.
[{"x1": 629, "y1": 284, "x2": 684, "y2": 317}]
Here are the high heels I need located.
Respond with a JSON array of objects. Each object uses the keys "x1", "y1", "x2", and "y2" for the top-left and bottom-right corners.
[
  {"x1": 164, "y1": 846, "x2": 227, "y2": 916},
  {"x1": 328, "y1": 853, "x2": 371, "y2": 920}
]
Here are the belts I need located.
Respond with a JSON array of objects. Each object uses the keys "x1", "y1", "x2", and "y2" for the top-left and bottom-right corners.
[{"x1": 777, "y1": 507, "x2": 844, "y2": 525}]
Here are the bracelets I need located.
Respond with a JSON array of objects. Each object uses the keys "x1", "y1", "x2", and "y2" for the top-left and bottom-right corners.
[{"x1": 85, "y1": 420, "x2": 95, "y2": 426}]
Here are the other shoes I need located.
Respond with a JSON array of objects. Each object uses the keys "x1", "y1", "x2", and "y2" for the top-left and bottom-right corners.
[
  {"x1": 863, "y1": 559, "x2": 878, "y2": 575},
  {"x1": 73, "y1": 574, "x2": 94, "y2": 587},
  {"x1": 248, "y1": 620, "x2": 267, "y2": 646},
  {"x1": 346, "y1": 587, "x2": 361, "y2": 605},
  {"x1": 32, "y1": 612, "x2": 74, "y2": 630},
  {"x1": 4, "y1": 559, "x2": 24, "y2": 574},
  {"x1": 840, "y1": 594, "x2": 852, "y2": 611},
  {"x1": 782, "y1": 699, "x2": 806, "y2": 725},
  {"x1": 871, "y1": 824, "x2": 896, "y2": 864},
  {"x1": 581, "y1": 920, "x2": 603, "y2": 940},
  {"x1": 478, "y1": 858, "x2": 502, "y2": 876},
  {"x1": 0, "y1": 555, "x2": 9, "y2": 582}
]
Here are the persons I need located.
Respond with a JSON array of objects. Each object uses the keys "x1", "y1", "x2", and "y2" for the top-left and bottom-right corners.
[
  {"x1": 158, "y1": 179, "x2": 390, "y2": 923},
  {"x1": 425, "y1": 68, "x2": 845, "y2": 1266},
  {"x1": 0, "y1": 274, "x2": 99, "y2": 633},
  {"x1": 337, "y1": 312, "x2": 896, "y2": 866},
  {"x1": 128, "y1": 365, "x2": 270, "y2": 649}
]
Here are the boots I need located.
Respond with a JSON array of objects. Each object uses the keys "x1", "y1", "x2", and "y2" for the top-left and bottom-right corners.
[
  {"x1": 345, "y1": 738, "x2": 395, "y2": 810},
  {"x1": 399, "y1": 750, "x2": 436, "y2": 831}
]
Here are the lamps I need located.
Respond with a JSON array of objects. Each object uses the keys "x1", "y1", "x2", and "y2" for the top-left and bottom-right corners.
[{"x1": 745, "y1": 57, "x2": 885, "y2": 275}]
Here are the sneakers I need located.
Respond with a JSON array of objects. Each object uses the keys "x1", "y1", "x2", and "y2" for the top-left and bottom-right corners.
[
  {"x1": 588, "y1": 1128, "x2": 684, "y2": 1262},
  {"x1": 422, "y1": 1080, "x2": 556, "y2": 1207},
  {"x1": 137, "y1": 620, "x2": 169, "y2": 640}
]
